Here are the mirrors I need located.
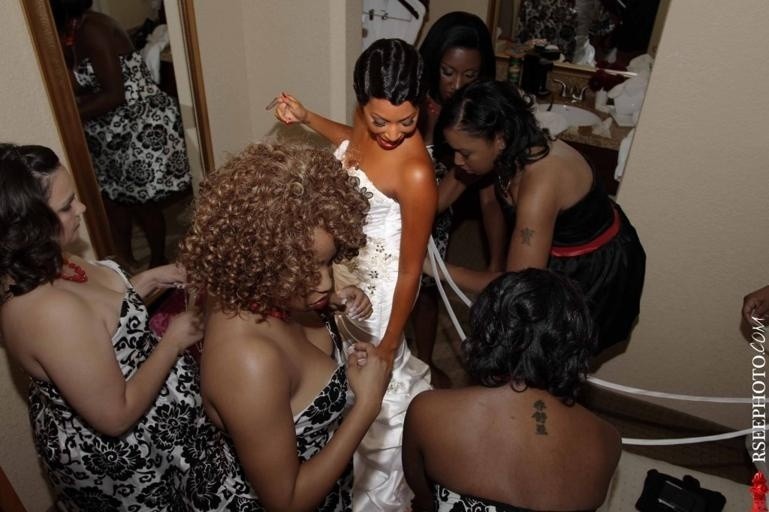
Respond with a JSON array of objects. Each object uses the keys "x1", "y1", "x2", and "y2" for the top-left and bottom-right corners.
[
  {"x1": 21, "y1": 0, "x2": 218, "y2": 283},
  {"x1": 484, "y1": 1, "x2": 670, "y2": 81}
]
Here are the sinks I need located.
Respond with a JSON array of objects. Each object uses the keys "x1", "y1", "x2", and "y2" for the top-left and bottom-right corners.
[{"x1": 538, "y1": 103, "x2": 601, "y2": 128}]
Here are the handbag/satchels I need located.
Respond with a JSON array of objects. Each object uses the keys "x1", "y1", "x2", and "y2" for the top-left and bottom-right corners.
[{"x1": 634, "y1": 468, "x2": 728, "y2": 512}]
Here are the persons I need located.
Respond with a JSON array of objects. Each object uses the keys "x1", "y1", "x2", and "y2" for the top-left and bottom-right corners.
[
  {"x1": 742, "y1": 285, "x2": 769, "y2": 326},
  {"x1": 0, "y1": 11, "x2": 647, "y2": 512},
  {"x1": 50, "y1": 0, "x2": 192, "y2": 270}
]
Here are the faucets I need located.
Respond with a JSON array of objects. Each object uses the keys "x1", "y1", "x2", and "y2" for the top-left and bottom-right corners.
[{"x1": 551, "y1": 76, "x2": 589, "y2": 102}]
